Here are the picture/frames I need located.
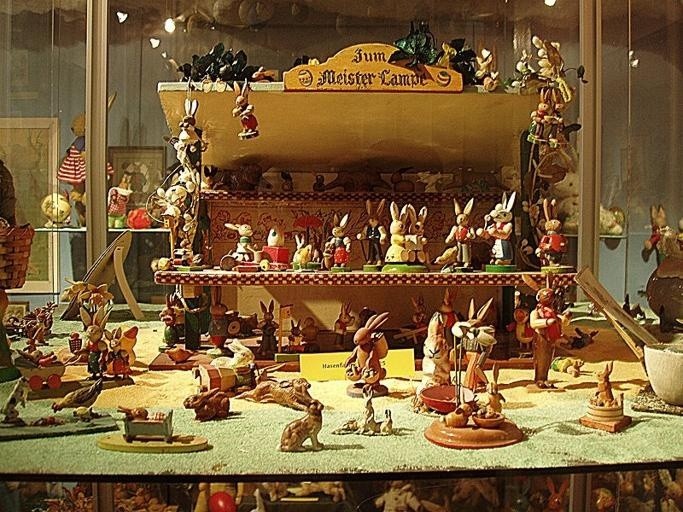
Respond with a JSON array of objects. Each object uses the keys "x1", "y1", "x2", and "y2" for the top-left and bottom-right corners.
[
  {"x1": 107, "y1": 146, "x2": 168, "y2": 209},
  {"x1": 0, "y1": 116, "x2": 61, "y2": 296}
]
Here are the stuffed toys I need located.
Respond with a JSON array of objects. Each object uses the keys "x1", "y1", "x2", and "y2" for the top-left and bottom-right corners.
[
  {"x1": 550, "y1": 170, "x2": 623, "y2": 235},
  {"x1": 58, "y1": 116, "x2": 114, "y2": 205}
]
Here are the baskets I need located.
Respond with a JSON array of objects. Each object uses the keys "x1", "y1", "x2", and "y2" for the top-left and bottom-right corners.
[{"x1": 0, "y1": 224, "x2": 33, "y2": 289}]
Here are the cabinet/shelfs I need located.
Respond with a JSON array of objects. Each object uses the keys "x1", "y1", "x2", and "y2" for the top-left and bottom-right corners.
[{"x1": 149, "y1": 41, "x2": 577, "y2": 373}]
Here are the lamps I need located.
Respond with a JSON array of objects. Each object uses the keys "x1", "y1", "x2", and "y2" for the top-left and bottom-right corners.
[{"x1": 112, "y1": 5, "x2": 183, "y2": 49}]
